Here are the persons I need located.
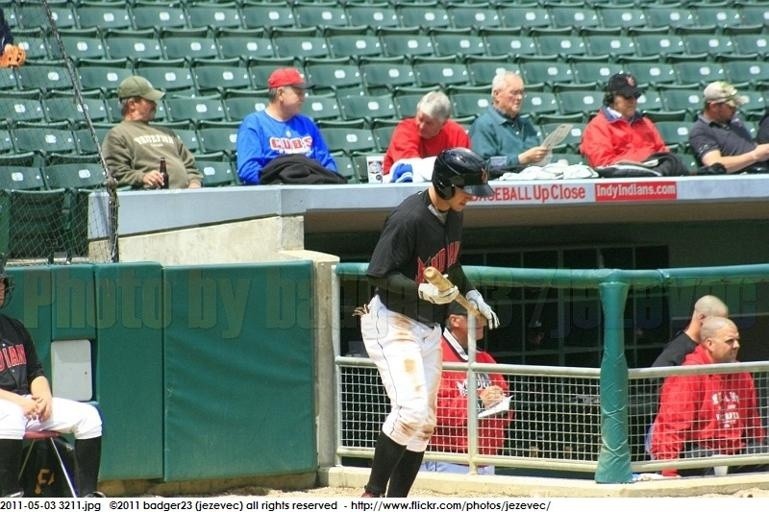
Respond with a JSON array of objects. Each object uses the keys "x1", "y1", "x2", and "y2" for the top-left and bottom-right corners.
[
  {"x1": 359, "y1": 146, "x2": 502, "y2": 498},
  {"x1": 649, "y1": 295, "x2": 729, "y2": 394},
  {"x1": 1, "y1": 251, "x2": 103, "y2": 497},
  {"x1": 651, "y1": 316, "x2": 766, "y2": 476},
  {"x1": 102, "y1": 76, "x2": 205, "y2": 191},
  {"x1": 236, "y1": 67, "x2": 338, "y2": 186},
  {"x1": 418, "y1": 300, "x2": 514, "y2": 475},
  {"x1": 0, "y1": 8, "x2": 27, "y2": 69},
  {"x1": 382, "y1": 67, "x2": 769, "y2": 176}
]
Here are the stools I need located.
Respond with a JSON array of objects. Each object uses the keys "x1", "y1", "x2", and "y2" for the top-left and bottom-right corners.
[{"x1": 5, "y1": 432, "x2": 78, "y2": 498}]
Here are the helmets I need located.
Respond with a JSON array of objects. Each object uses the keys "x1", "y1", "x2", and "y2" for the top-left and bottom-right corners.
[{"x1": 431, "y1": 147, "x2": 495, "y2": 201}]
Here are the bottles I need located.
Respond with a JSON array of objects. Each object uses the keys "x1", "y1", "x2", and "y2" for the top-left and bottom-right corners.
[{"x1": 157, "y1": 155, "x2": 170, "y2": 190}]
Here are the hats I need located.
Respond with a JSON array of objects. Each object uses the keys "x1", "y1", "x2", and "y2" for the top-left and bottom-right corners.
[
  {"x1": 117, "y1": 75, "x2": 166, "y2": 101},
  {"x1": 608, "y1": 73, "x2": 644, "y2": 98},
  {"x1": 703, "y1": 80, "x2": 746, "y2": 109},
  {"x1": 267, "y1": 67, "x2": 316, "y2": 90}
]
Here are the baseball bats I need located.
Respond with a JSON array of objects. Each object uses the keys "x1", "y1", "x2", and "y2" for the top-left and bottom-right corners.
[{"x1": 423, "y1": 267, "x2": 489, "y2": 326}]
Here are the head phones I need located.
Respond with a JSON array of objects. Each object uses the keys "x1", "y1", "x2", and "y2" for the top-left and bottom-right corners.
[{"x1": 602, "y1": 73, "x2": 619, "y2": 106}]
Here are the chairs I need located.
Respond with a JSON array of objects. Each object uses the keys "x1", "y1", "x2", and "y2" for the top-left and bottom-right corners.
[{"x1": 0, "y1": 0, "x2": 769, "y2": 257}]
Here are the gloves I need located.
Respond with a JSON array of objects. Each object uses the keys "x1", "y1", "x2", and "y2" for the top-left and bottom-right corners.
[
  {"x1": 418, "y1": 274, "x2": 459, "y2": 305},
  {"x1": 466, "y1": 288, "x2": 501, "y2": 330}
]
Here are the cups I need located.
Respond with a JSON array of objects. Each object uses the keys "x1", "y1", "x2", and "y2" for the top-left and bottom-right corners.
[
  {"x1": 710, "y1": 454, "x2": 730, "y2": 477},
  {"x1": 364, "y1": 155, "x2": 386, "y2": 185}
]
